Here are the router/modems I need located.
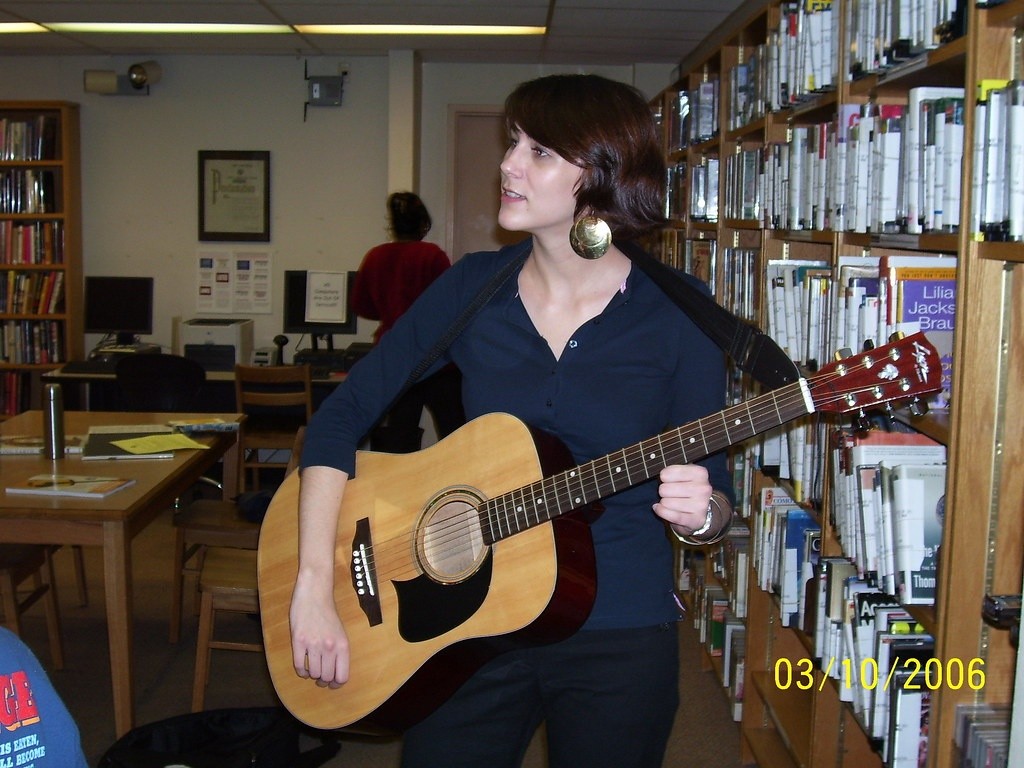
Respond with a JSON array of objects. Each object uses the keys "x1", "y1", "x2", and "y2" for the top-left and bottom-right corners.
[{"x1": 303, "y1": 59, "x2": 342, "y2": 122}]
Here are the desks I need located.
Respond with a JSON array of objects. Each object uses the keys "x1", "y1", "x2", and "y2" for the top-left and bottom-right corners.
[
  {"x1": 43, "y1": 358, "x2": 350, "y2": 501},
  {"x1": 1, "y1": 411, "x2": 244, "y2": 740}
]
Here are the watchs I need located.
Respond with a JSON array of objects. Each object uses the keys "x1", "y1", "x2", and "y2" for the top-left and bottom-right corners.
[{"x1": 692, "y1": 504, "x2": 712, "y2": 536}]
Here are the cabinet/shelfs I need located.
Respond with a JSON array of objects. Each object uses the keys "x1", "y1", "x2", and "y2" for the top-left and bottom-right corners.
[
  {"x1": 649, "y1": 1, "x2": 1024, "y2": 768},
  {"x1": 0, "y1": 100, "x2": 83, "y2": 422}
]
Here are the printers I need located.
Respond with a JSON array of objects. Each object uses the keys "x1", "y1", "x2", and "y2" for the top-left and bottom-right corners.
[{"x1": 181, "y1": 318, "x2": 254, "y2": 372}]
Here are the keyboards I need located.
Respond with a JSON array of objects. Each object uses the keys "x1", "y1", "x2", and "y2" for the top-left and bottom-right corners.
[
  {"x1": 309, "y1": 367, "x2": 331, "y2": 379},
  {"x1": 61, "y1": 360, "x2": 121, "y2": 373}
]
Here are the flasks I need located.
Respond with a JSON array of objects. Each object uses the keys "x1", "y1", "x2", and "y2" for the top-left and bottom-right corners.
[{"x1": 41, "y1": 383, "x2": 66, "y2": 460}]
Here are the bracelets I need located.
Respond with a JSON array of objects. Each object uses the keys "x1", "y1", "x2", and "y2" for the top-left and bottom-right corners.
[
  {"x1": 688, "y1": 493, "x2": 733, "y2": 544},
  {"x1": 670, "y1": 498, "x2": 723, "y2": 545}
]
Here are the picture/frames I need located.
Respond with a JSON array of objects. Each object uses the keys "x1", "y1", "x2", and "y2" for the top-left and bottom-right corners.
[{"x1": 199, "y1": 148, "x2": 272, "y2": 242}]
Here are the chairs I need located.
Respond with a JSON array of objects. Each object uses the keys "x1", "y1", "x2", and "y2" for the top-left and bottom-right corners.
[{"x1": 0, "y1": 366, "x2": 311, "y2": 714}]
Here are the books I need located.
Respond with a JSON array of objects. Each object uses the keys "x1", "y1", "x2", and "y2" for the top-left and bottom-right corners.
[
  {"x1": 0, "y1": 424, "x2": 173, "y2": 498},
  {"x1": 0, "y1": 116, "x2": 62, "y2": 415}
]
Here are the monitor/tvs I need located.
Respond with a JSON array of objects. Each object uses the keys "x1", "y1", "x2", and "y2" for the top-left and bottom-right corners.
[
  {"x1": 283, "y1": 270, "x2": 357, "y2": 357},
  {"x1": 84, "y1": 276, "x2": 153, "y2": 349}
]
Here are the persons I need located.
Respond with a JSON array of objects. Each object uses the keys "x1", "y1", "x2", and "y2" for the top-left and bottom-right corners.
[
  {"x1": 0, "y1": 626, "x2": 86, "y2": 767},
  {"x1": 289, "y1": 76, "x2": 736, "y2": 767},
  {"x1": 660, "y1": 0, "x2": 1024, "y2": 768},
  {"x1": 351, "y1": 193, "x2": 464, "y2": 441}
]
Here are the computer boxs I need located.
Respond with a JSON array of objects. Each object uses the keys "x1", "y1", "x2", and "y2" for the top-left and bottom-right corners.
[
  {"x1": 293, "y1": 348, "x2": 348, "y2": 372},
  {"x1": 89, "y1": 345, "x2": 163, "y2": 362}
]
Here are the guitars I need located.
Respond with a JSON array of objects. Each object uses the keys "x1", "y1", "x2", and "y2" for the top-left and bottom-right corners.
[{"x1": 254, "y1": 329, "x2": 945, "y2": 741}]
